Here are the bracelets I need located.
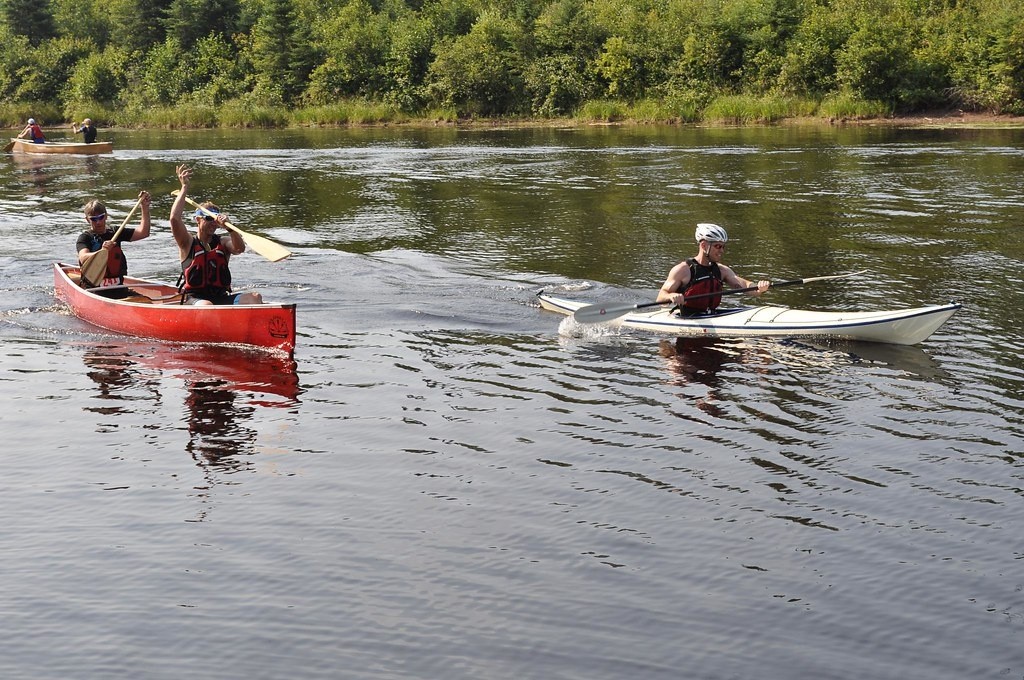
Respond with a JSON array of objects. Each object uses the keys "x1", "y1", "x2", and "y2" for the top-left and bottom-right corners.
[{"x1": 226, "y1": 224, "x2": 237, "y2": 233}]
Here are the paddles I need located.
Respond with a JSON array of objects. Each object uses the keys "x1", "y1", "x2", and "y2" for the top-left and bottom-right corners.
[
  {"x1": 2, "y1": 122, "x2": 32, "y2": 154},
  {"x1": 78, "y1": 192, "x2": 149, "y2": 293},
  {"x1": 168, "y1": 188, "x2": 295, "y2": 265},
  {"x1": 566, "y1": 268, "x2": 863, "y2": 324}
]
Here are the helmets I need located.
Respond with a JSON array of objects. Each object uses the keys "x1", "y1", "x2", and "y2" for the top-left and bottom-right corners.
[{"x1": 695, "y1": 223, "x2": 728, "y2": 242}]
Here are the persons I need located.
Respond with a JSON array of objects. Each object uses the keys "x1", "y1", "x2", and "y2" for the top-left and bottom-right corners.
[
  {"x1": 169, "y1": 163, "x2": 262, "y2": 306},
  {"x1": 76, "y1": 190, "x2": 151, "y2": 299},
  {"x1": 656, "y1": 223, "x2": 770, "y2": 317},
  {"x1": 72, "y1": 118, "x2": 97, "y2": 143},
  {"x1": 17, "y1": 118, "x2": 45, "y2": 144}
]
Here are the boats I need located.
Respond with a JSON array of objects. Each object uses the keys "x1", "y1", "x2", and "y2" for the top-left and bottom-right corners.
[
  {"x1": 52, "y1": 259, "x2": 298, "y2": 358},
  {"x1": 10, "y1": 137, "x2": 113, "y2": 155},
  {"x1": 537, "y1": 289, "x2": 964, "y2": 347}
]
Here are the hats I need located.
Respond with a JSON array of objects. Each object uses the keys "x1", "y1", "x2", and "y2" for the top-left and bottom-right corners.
[
  {"x1": 28, "y1": 119, "x2": 34, "y2": 124},
  {"x1": 82, "y1": 119, "x2": 91, "y2": 124}
]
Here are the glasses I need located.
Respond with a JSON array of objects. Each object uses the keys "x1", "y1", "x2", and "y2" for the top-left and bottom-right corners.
[
  {"x1": 712, "y1": 244, "x2": 725, "y2": 249},
  {"x1": 88, "y1": 213, "x2": 106, "y2": 222},
  {"x1": 195, "y1": 215, "x2": 213, "y2": 221}
]
